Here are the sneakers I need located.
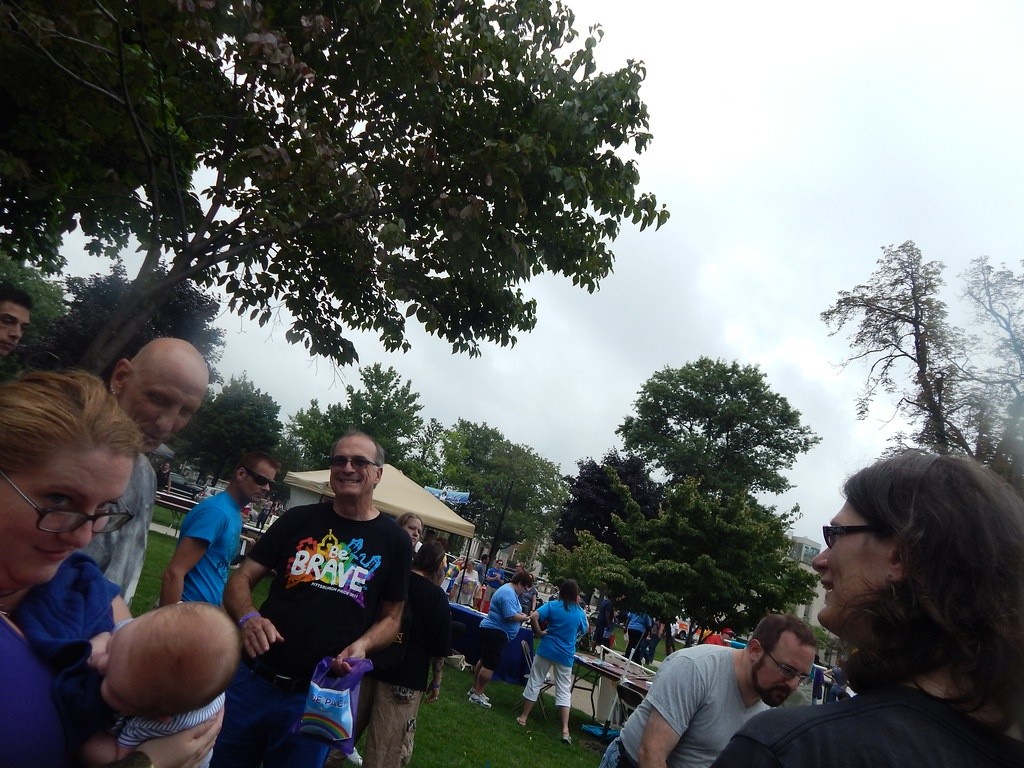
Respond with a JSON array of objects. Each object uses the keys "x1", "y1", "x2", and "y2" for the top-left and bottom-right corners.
[
  {"x1": 466, "y1": 686, "x2": 490, "y2": 702},
  {"x1": 468, "y1": 693, "x2": 493, "y2": 709}
]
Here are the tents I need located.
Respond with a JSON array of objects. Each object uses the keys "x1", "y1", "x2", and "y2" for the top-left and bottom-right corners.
[{"x1": 283, "y1": 463, "x2": 475, "y2": 603}]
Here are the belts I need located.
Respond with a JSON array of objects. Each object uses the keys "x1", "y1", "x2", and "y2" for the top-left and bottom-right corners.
[{"x1": 615, "y1": 736, "x2": 639, "y2": 768}]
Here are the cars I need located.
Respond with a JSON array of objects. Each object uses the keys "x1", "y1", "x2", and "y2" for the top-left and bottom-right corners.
[
  {"x1": 169, "y1": 471, "x2": 204, "y2": 495},
  {"x1": 196, "y1": 486, "x2": 260, "y2": 525},
  {"x1": 674, "y1": 613, "x2": 701, "y2": 644}
]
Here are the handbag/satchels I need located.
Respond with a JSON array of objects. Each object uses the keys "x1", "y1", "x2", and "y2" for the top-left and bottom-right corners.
[
  {"x1": 291, "y1": 656, "x2": 374, "y2": 755},
  {"x1": 603, "y1": 627, "x2": 617, "y2": 655},
  {"x1": 623, "y1": 626, "x2": 629, "y2": 645},
  {"x1": 522, "y1": 605, "x2": 531, "y2": 614}
]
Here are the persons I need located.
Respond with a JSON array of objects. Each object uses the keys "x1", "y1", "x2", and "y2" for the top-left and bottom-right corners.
[
  {"x1": 440, "y1": 492, "x2": 447, "y2": 500},
  {"x1": 0, "y1": 274, "x2": 449, "y2": 768},
  {"x1": 449, "y1": 554, "x2": 589, "y2": 744},
  {"x1": 709, "y1": 448, "x2": 1024, "y2": 768},
  {"x1": 592, "y1": 593, "x2": 847, "y2": 706},
  {"x1": 599, "y1": 614, "x2": 817, "y2": 768}
]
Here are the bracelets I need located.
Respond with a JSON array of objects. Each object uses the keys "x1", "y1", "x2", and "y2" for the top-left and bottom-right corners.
[{"x1": 237, "y1": 612, "x2": 260, "y2": 626}]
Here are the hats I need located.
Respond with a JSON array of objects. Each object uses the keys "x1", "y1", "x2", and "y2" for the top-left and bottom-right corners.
[{"x1": 721, "y1": 627, "x2": 735, "y2": 634}]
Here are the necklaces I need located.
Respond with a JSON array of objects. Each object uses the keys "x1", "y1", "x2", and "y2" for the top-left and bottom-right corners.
[{"x1": 432, "y1": 681, "x2": 439, "y2": 688}]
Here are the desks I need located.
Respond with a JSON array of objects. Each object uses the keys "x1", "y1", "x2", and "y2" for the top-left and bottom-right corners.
[
  {"x1": 155, "y1": 486, "x2": 267, "y2": 555},
  {"x1": 571, "y1": 652, "x2": 652, "y2": 741},
  {"x1": 449, "y1": 602, "x2": 540, "y2": 686}
]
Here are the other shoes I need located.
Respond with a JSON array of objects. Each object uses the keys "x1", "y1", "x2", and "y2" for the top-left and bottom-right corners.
[{"x1": 344, "y1": 745, "x2": 364, "y2": 766}]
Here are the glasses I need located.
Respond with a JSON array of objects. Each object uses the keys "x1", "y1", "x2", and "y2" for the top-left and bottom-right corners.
[
  {"x1": 0, "y1": 468, "x2": 136, "y2": 534},
  {"x1": 236, "y1": 465, "x2": 276, "y2": 490},
  {"x1": 497, "y1": 562, "x2": 503, "y2": 566},
  {"x1": 759, "y1": 642, "x2": 809, "y2": 688},
  {"x1": 522, "y1": 585, "x2": 529, "y2": 592},
  {"x1": 328, "y1": 454, "x2": 378, "y2": 469},
  {"x1": 822, "y1": 524, "x2": 885, "y2": 549}
]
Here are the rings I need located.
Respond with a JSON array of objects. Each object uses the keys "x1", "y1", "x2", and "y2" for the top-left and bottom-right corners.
[{"x1": 435, "y1": 697, "x2": 438, "y2": 700}]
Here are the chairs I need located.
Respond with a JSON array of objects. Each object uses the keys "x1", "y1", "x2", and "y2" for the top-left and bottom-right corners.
[
  {"x1": 616, "y1": 685, "x2": 643, "y2": 721},
  {"x1": 511, "y1": 640, "x2": 556, "y2": 724}
]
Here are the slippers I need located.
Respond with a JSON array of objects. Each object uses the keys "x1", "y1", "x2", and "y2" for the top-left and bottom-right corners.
[
  {"x1": 516, "y1": 716, "x2": 527, "y2": 726},
  {"x1": 561, "y1": 733, "x2": 573, "y2": 746}
]
